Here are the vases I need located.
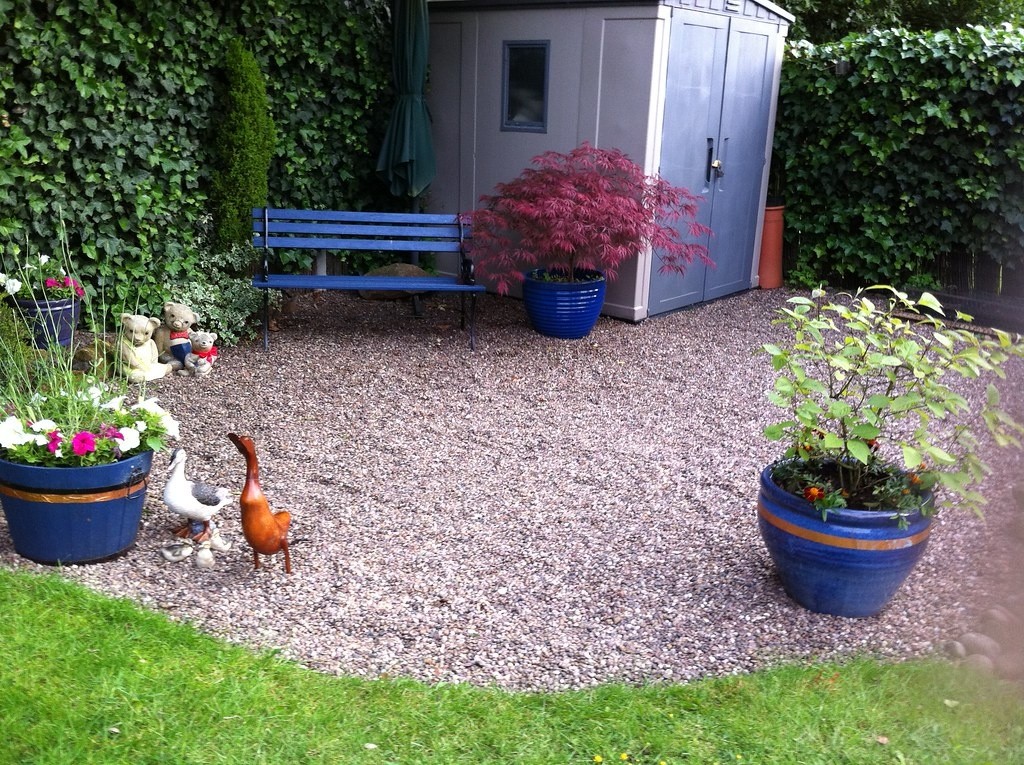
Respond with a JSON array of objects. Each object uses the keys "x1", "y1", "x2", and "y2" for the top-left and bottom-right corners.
[
  {"x1": 0, "y1": 448, "x2": 154, "y2": 567},
  {"x1": 6, "y1": 296, "x2": 81, "y2": 350}
]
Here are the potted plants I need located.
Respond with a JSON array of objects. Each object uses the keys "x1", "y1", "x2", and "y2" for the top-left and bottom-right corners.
[
  {"x1": 756, "y1": 281, "x2": 1024, "y2": 619},
  {"x1": 451, "y1": 139, "x2": 719, "y2": 340}
]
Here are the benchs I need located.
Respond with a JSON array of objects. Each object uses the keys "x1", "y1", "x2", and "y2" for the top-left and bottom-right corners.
[{"x1": 250, "y1": 206, "x2": 489, "y2": 351}]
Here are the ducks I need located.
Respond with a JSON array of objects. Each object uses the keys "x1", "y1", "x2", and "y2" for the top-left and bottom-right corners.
[
  {"x1": 162, "y1": 448, "x2": 233, "y2": 545},
  {"x1": 228, "y1": 433, "x2": 293, "y2": 574}
]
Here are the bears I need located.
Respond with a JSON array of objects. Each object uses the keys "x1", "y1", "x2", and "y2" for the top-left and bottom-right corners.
[{"x1": 114, "y1": 302, "x2": 219, "y2": 383}]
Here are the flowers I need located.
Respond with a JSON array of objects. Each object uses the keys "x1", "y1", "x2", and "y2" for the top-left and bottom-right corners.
[
  {"x1": 0, "y1": 380, "x2": 181, "y2": 466},
  {"x1": 0, "y1": 255, "x2": 85, "y2": 300}
]
